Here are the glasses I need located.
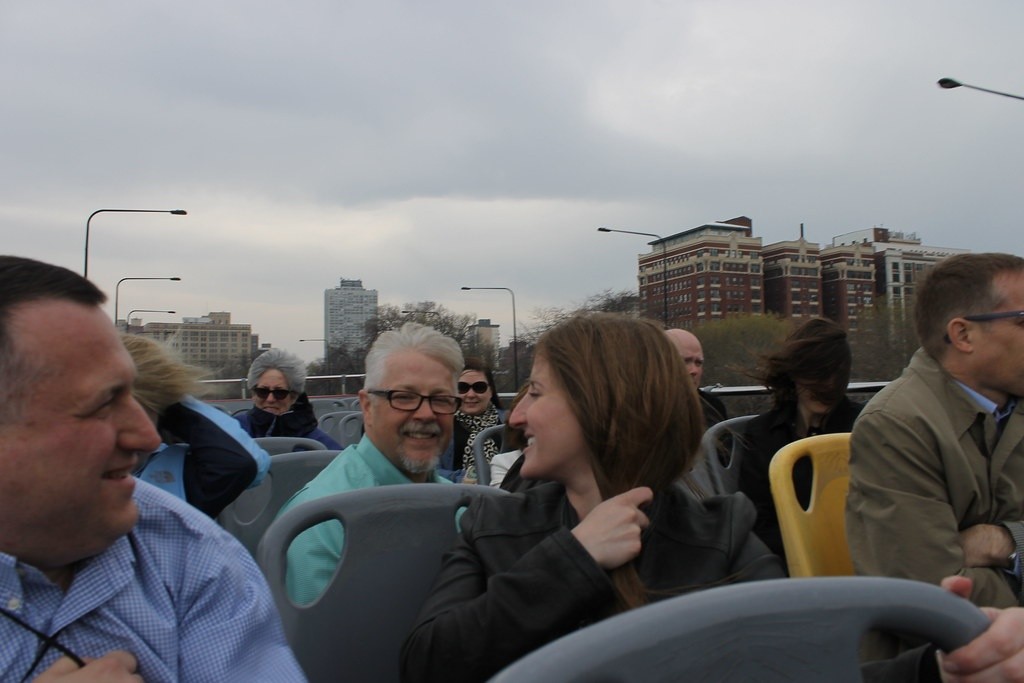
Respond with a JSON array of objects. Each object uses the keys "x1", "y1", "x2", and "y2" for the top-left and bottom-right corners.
[
  {"x1": 458, "y1": 381, "x2": 489, "y2": 394},
  {"x1": 252, "y1": 387, "x2": 294, "y2": 400},
  {"x1": 367, "y1": 389, "x2": 463, "y2": 415}
]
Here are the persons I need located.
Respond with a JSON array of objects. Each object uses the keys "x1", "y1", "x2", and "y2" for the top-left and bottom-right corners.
[
  {"x1": 396, "y1": 312, "x2": 1024, "y2": 683},
  {"x1": 229, "y1": 349, "x2": 346, "y2": 450},
  {"x1": 741, "y1": 316, "x2": 866, "y2": 579},
  {"x1": 122, "y1": 333, "x2": 271, "y2": 525},
  {"x1": 274, "y1": 323, "x2": 468, "y2": 604},
  {"x1": 844, "y1": 252, "x2": 1024, "y2": 609},
  {"x1": 436, "y1": 329, "x2": 733, "y2": 493},
  {"x1": 0, "y1": 256, "x2": 312, "y2": 683}
]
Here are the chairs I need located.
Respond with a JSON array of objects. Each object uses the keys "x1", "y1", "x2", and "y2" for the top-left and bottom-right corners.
[{"x1": 211, "y1": 392, "x2": 990, "y2": 683}]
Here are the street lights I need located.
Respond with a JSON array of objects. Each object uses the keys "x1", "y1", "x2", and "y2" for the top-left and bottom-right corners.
[
  {"x1": 127, "y1": 310, "x2": 175, "y2": 333},
  {"x1": 461, "y1": 287, "x2": 518, "y2": 391},
  {"x1": 83, "y1": 209, "x2": 187, "y2": 280},
  {"x1": 116, "y1": 278, "x2": 181, "y2": 331},
  {"x1": 401, "y1": 310, "x2": 441, "y2": 331},
  {"x1": 300, "y1": 339, "x2": 331, "y2": 394},
  {"x1": 598, "y1": 228, "x2": 668, "y2": 330}
]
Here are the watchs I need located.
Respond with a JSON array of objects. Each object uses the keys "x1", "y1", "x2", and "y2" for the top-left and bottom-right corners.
[{"x1": 1004, "y1": 545, "x2": 1017, "y2": 571}]
